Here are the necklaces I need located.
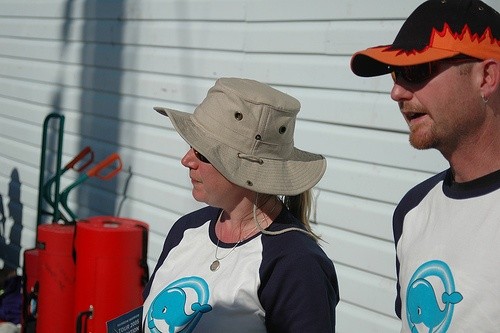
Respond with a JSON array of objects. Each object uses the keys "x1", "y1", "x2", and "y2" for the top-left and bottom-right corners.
[{"x1": 209, "y1": 200, "x2": 280, "y2": 272}]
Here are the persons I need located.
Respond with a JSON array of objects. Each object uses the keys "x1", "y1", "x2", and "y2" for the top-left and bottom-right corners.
[
  {"x1": 142, "y1": 78, "x2": 340, "y2": 333},
  {"x1": 350, "y1": 0, "x2": 500, "y2": 333}
]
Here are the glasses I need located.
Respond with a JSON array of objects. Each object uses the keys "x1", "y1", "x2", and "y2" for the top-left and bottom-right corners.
[
  {"x1": 387, "y1": 56, "x2": 479, "y2": 84},
  {"x1": 190, "y1": 145, "x2": 210, "y2": 163}
]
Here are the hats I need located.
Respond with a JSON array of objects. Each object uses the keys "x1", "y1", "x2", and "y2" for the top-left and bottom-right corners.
[
  {"x1": 350, "y1": 0, "x2": 500, "y2": 78},
  {"x1": 153, "y1": 77, "x2": 327, "y2": 196}
]
